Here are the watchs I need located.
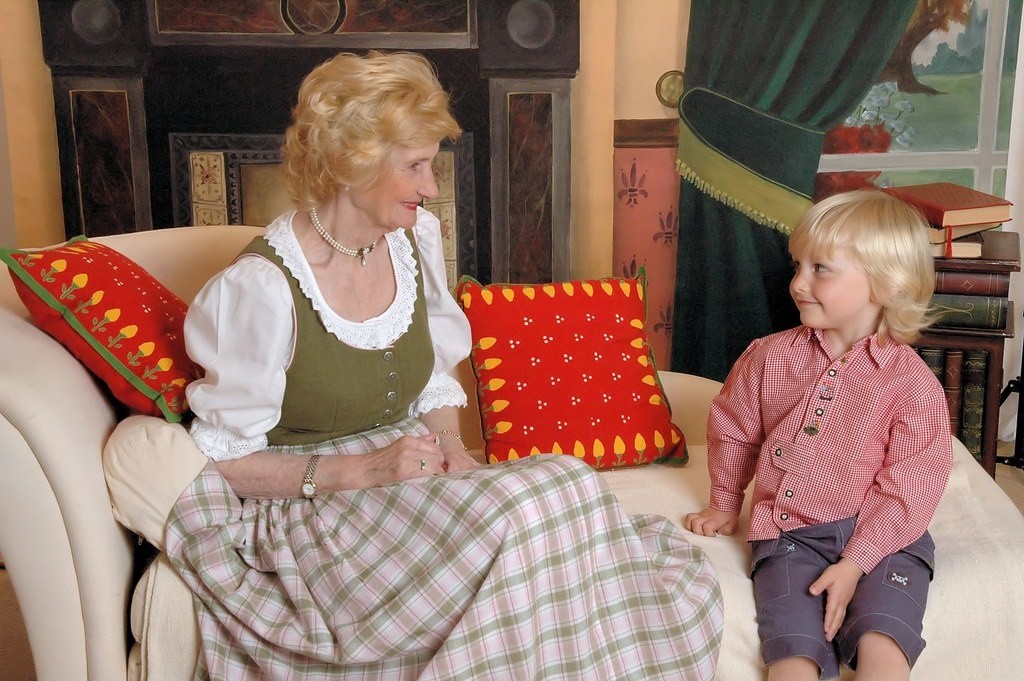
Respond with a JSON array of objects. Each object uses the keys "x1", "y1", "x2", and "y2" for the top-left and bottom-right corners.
[{"x1": 300, "y1": 455, "x2": 318, "y2": 498}]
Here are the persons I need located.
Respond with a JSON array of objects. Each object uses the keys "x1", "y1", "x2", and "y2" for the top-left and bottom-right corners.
[
  {"x1": 687, "y1": 189, "x2": 952, "y2": 680},
  {"x1": 164, "y1": 48, "x2": 726, "y2": 681}
]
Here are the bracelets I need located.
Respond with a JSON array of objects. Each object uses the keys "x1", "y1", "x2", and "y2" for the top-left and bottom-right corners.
[{"x1": 435, "y1": 431, "x2": 466, "y2": 449}]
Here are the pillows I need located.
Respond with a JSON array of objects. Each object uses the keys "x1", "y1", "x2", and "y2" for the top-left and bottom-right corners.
[
  {"x1": 453, "y1": 266, "x2": 695, "y2": 471},
  {"x1": 0, "y1": 232, "x2": 209, "y2": 427}
]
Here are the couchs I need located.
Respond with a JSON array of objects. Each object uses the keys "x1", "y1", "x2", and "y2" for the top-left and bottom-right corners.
[{"x1": 2, "y1": 225, "x2": 1024, "y2": 679}]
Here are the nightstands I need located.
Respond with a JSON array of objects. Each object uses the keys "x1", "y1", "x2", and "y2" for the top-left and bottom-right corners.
[{"x1": 911, "y1": 230, "x2": 1020, "y2": 482}]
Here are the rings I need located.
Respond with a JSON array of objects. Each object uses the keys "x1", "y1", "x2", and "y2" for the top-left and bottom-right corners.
[{"x1": 420, "y1": 459, "x2": 426, "y2": 470}]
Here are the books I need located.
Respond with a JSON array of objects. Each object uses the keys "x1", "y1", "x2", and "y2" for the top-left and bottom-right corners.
[
  {"x1": 924, "y1": 293, "x2": 1007, "y2": 330},
  {"x1": 945, "y1": 349, "x2": 962, "y2": 437},
  {"x1": 919, "y1": 347, "x2": 944, "y2": 380},
  {"x1": 934, "y1": 233, "x2": 983, "y2": 258},
  {"x1": 921, "y1": 225, "x2": 1004, "y2": 245},
  {"x1": 933, "y1": 271, "x2": 1010, "y2": 297},
  {"x1": 884, "y1": 182, "x2": 1012, "y2": 224},
  {"x1": 964, "y1": 352, "x2": 988, "y2": 462}
]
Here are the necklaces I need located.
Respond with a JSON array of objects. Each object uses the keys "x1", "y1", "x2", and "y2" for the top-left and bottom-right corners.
[{"x1": 308, "y1": 203, "x2": 375, "y2": 267}]
